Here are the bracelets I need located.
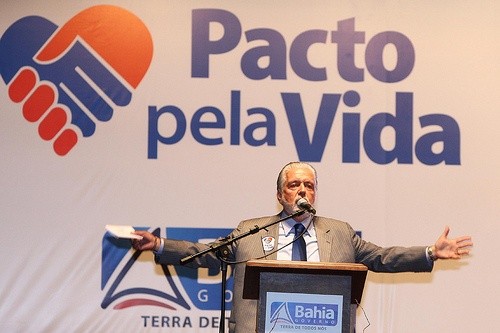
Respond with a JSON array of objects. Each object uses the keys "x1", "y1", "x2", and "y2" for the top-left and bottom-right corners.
[
  {"x1": 151, "y1": 236, "x2": 158, "y2": 251},
  {"x1": 427, "y1": 245, "x2": 436, "y2": 260}
]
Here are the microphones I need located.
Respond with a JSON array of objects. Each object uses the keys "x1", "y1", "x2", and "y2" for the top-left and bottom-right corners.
[{"x1": 297, "y1": 198, "x2": 316, "y2": 214}]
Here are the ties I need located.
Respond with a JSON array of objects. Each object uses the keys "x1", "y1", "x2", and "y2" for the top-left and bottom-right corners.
[{"x1": 291, "y1": 223, "x2": 307, "y2": 262}]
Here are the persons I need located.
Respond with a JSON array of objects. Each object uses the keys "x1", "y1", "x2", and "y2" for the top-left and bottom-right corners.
[{"x1": 130, "y1": 161, "x2": 473, "y2": 333}]
